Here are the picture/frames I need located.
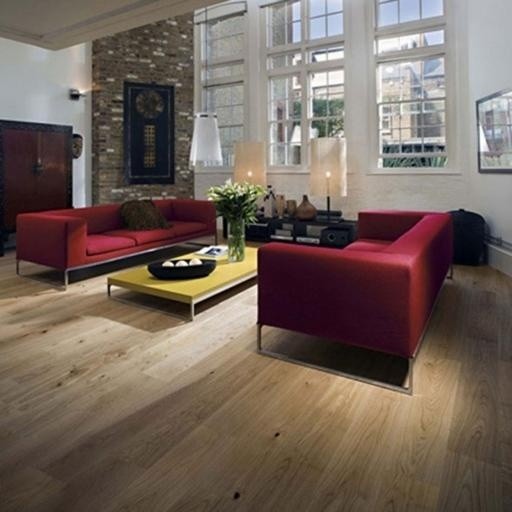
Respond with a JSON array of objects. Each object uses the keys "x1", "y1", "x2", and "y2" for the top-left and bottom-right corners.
[{"x1": 474, "y1": 86, "x2": 512, "y2": 174}]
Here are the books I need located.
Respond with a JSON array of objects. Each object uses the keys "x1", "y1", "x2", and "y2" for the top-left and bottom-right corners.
[{"x1": 195, "y1": 243, "x2": 229, "y2": 258}]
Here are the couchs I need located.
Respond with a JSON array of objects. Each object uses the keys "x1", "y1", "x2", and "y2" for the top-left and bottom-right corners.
[
  {"x1": 257, "y1": 209, "x2": 452, "y2": 397},
  {"x1": 16, "y1": 198, "x2": 217, "y2": 290}
]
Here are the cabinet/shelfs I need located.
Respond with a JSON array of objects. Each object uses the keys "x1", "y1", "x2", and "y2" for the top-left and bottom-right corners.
[{"x1": 2, "y1": 120, "x2": 74, "y2": 258}]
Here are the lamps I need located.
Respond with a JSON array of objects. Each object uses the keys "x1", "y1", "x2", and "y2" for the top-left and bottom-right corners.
[
  {"x1": 234, "y1": 140, "x2": 267, "y2": 188},
  {"x1": 188, "y1": 1, "x2": 224, "y2": 168},
  {"x1": 310, "y1": 136, "x2": 346, "y2": 222}
]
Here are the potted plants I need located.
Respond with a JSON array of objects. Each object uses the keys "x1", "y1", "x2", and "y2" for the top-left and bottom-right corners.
[{"x1": 208, "y1": 180, "x2": 262, "y2": 261}]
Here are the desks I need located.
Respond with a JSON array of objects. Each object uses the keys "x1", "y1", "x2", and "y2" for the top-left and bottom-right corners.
[{"x1": 108, "y1": 245, "x2": 259, "y2": 320}]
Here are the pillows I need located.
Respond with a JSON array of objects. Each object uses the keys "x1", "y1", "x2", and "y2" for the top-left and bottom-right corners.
[{"x1": 120, "y1": 200, "x2": 174, "y2": 229}]
